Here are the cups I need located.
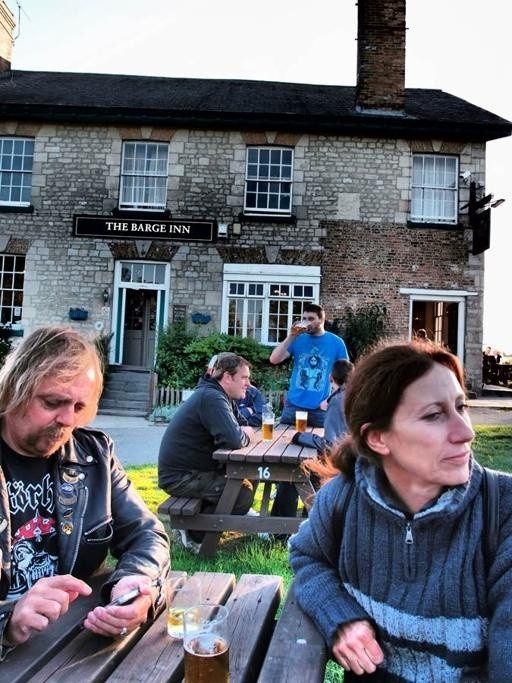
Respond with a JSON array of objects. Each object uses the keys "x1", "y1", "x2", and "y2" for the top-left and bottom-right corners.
[
  {"x1": 296, "y1": 411, "x2": 308, "y2": 432},
  {"x1": 165, "y1": 576, "x2": 229, "y2": 683},
  {"x1": 262, "y1": 413, "x2": 275, "y2": 441},
  {"x1": 290, "y1": 320, "x2": 310, "y2": 337}
]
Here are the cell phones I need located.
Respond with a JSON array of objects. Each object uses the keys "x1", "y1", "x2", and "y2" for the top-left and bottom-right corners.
[{"x1": 79, "y1": 587, "x2": 141, "y2": 630}]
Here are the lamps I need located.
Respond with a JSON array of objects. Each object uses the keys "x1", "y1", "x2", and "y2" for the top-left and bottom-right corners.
[{"x1": 102, "y1": 290, "x2": 108, "y2": 302}]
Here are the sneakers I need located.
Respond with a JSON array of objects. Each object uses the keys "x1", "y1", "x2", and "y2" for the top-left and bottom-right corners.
[
  {"x1": 172, "y1": 529, "x2": 202, "y2": 555},
  {"x1": 268, "y1": 489, "x2": 277, "y2": 501}
]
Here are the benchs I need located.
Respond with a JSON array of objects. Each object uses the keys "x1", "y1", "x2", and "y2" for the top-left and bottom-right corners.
[{"x1": 158, "y1": 495, "x2": 203, "y2": 531}]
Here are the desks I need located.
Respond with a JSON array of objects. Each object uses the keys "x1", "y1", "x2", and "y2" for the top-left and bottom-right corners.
[
  {"x1": 1, "y1": 565, "x2": 331, "y2": 681},
  {"x1": 0, "y1": 421, "x2": 332, "y2": 683},
  {"x1": 197, "y1": 423, "x2": 332, "y2": 562}
]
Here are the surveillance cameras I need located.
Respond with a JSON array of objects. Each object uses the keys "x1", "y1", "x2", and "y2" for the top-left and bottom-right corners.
[{"x1": 459, "y1": 171, "x2": 471, "y2": 180}]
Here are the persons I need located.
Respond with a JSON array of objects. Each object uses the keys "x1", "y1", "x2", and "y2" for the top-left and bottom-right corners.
[
  {"x1": 0, "y1": 323, "x2": 175, "y2": 663},
  {"x1": 482, "y1": 346, "x2": 501, "y2": 388},
  {"x1": 416, "y1": 328, "x2": 431, "y2": 343},
  {"x1": 157, "y1": 353, "x2": 256, "y2": 557},
  {"x1": 301, "y1": 356, "x2": 322, "y2": 389},
  {"x1": 206, "y1": 350, "x2": 269, "y2": 542},
  {"x1": 286, "y1": 332, "x2": 512, "y2": 683},
  {"x1": 256, "y1": 305, "x2": 350, "y2": 542},
  {"x1": 290, "y1": 358, "x2": 354, "y2": 452}
]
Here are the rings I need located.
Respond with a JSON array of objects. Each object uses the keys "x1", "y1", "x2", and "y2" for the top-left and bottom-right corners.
[{"x1": 120, "y1": 627, "x2": 127, "y2": 637}]
